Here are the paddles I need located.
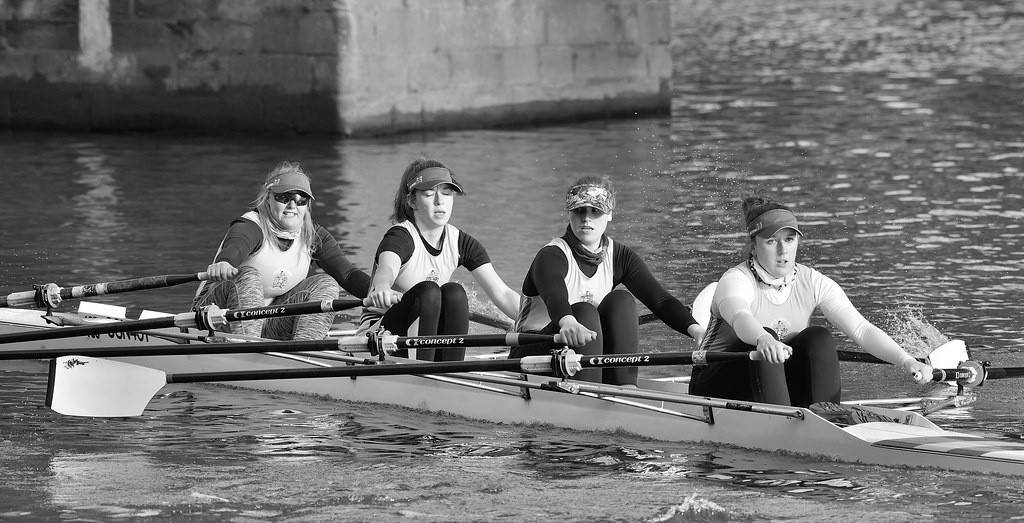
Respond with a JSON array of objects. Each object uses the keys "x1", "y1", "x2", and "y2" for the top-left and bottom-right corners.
[
  {"x1": 842, "y1": 338, "x2": 973, "y2": 372},
  {"x1": 0, "y1": 295, "x2": 367, "y2": 345},
  {"x1": 467, "y1": 307, "x2": 511, "y2": 329},
  {"x1": 1, "y1": 331, "x2": 568, "y2": 363},
  {"x1": 44, "y1": 348, "x2": 771, "y2": 419},
  {"x1": 640, "y1": 282, "x2": 717, "y2": 331},
  {"x1": 0, "y1": 268, "x2": 209, "y2": 309}
]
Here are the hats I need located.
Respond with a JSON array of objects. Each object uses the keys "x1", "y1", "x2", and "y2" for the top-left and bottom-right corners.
[
  {"x1": 263, "y1": 171, "x2": 315, "y2": 200},
  {"x1": 407, "y1": 166, "x2": 463, "y2": 196},
  {"x1": 747, "y1": 208, "x2": 804, "y2": 240},
  {"x1": 564, "y1": 183, "x2": 617, "y2": 215}
]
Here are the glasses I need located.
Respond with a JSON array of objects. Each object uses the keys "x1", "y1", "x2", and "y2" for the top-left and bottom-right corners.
[{"x1": 272, "y1": 192, "x2": 310, "y2": 206}]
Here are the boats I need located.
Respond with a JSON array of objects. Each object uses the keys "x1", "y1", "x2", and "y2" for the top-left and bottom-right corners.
[{"x1": 0, "y1": 298, "x2": 1023, "y2": 478}]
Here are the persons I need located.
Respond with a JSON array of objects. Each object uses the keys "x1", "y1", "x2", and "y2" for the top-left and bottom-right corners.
[
  {"x1": 689, "y1": 197, "x2": 933, "y2": 407},
  {"x1": 192, "y1": 164, "x2": 371, "y2": 340},
  {"x1": 353, "y1": 157, "x2": 520, "y2": 362},
  {"x1": 508, "y1": 175, "x2": 705, "y2": 393}
]
